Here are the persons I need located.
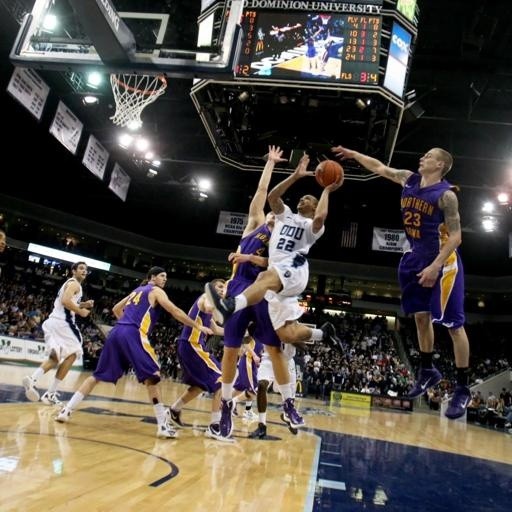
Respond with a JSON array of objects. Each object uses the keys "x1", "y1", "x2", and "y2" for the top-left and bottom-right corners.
[
  {"x1": 204, "y1": 153, "x2": 344, "y2": 352},
  {"x1": 0, "y1": 229, "x2": 511, "y2": 441},
  {"x1": 216, "y1": 144, "x2": 304, "y2": 438},
  {"x1": 254, "y1": 14, "x2": 341, "y2": 85},
  {"x1": 329, "y1": 143, "x2": 471, "y2": 418}
]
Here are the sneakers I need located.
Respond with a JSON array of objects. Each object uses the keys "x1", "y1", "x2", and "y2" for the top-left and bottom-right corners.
[
  {"x1": 408, "y1": 366, "x2": 442, "y2": 399},
  {"x1": 21, "y1": 375, "x2": 305, "y2": 439},
  {"x1": 444, "y1": 386, "x2": 472, "y2": 419}
]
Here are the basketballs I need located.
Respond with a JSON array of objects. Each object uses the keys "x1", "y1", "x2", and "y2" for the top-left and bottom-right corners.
[{"x1": 316, "y1": 160, "x2": 344, "y2": 187}]
